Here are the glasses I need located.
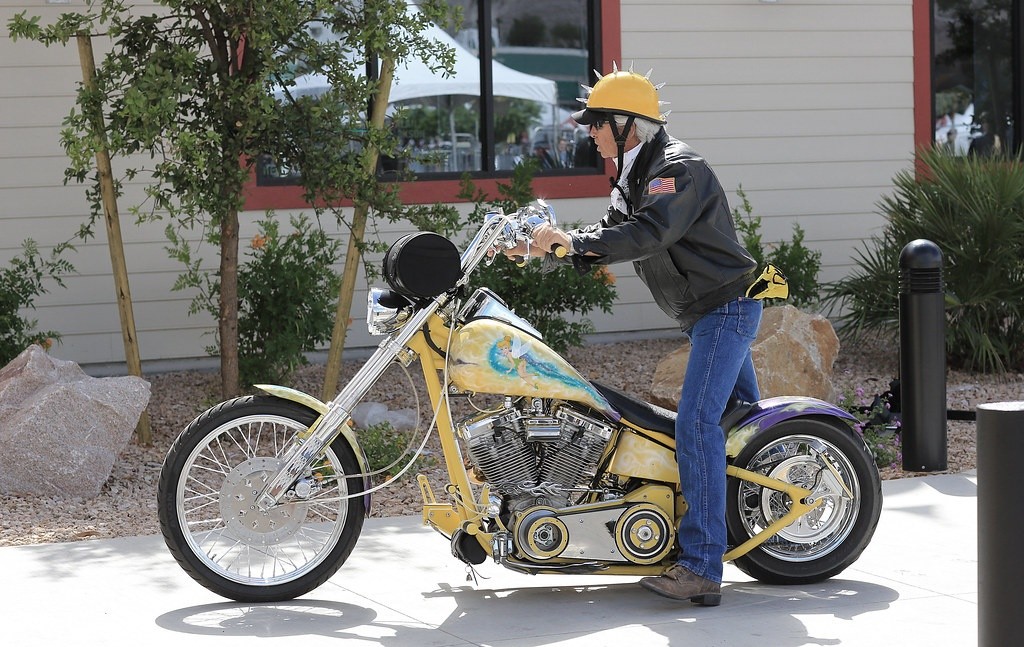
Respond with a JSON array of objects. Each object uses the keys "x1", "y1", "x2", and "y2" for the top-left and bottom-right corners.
[{"x1": 589, "y1": 120, "x2": 617, "y2": 131}]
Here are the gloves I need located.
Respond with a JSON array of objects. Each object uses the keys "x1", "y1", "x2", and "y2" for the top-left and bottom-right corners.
[{"x1": 745, "y1": 262, "x2": 789, "y2": 300}]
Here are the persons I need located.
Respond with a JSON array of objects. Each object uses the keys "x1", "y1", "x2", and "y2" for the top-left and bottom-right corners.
[
  {"x1": 487, "y1": 60, "x2": 762, "y2": 605},
  {"x1": 559, "y1": 139, "x2": 568, "y2": 162}
]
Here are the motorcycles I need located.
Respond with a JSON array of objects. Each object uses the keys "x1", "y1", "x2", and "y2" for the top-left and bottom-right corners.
[{"x1": 157, "y1": 198, "x2": 884, "y2": 603}]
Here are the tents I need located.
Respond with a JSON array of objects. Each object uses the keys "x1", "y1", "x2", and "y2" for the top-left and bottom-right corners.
[{"x1": 264, "y1": 0, "x2": 559, "y2": 170}]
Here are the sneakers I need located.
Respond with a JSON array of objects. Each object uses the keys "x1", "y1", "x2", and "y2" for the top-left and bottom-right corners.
[{"x1": 638, "y1": 563, "x2": 722, "y2": 606}]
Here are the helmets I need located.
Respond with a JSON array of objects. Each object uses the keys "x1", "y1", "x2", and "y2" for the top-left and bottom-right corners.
[{"x1": 571, "y1": 59, "x2": 672, "y2": 125}]
